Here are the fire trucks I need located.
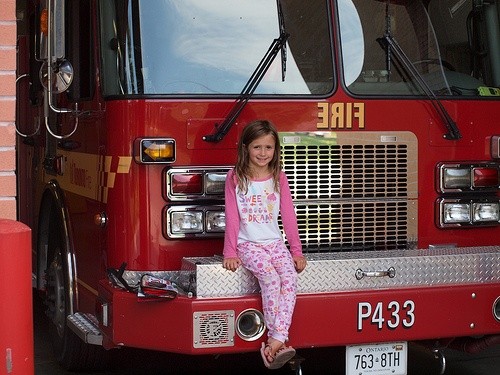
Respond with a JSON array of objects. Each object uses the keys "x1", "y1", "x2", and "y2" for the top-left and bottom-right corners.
[{"x1": 17, "y1": 0, "x2": 499, "y2": 351}]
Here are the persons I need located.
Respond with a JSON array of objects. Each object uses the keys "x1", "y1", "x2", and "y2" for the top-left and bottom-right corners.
[{"x1": 223, "y1": 120, "x2": 307, "y2": 369}]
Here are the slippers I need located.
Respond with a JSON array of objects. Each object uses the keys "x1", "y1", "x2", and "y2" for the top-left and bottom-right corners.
[
  {"x1": 260, "y1": 341, "x2": 274, "y2": 368},
  {"x1": 270, "y1": 347, "x2": 296, "y2": 369}
]
